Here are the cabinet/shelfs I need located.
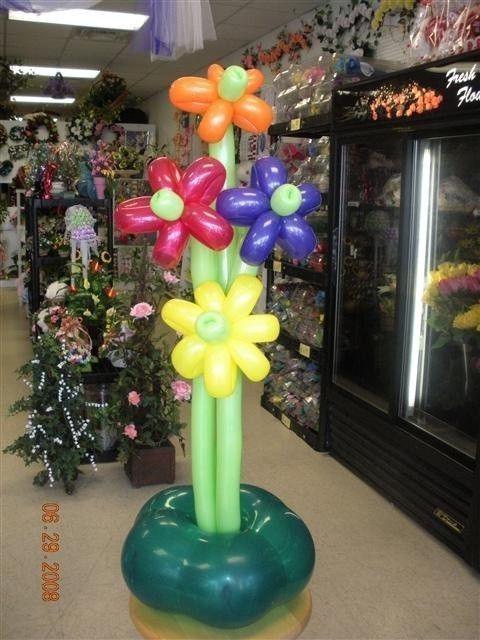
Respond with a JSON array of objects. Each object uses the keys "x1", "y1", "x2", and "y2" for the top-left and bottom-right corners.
[
  {"x1": 261, "y1": 113, "x2": 331, "y2": 453},
  {"x1": 23, "y1": 192, "x2": 114, "y2": 313}
]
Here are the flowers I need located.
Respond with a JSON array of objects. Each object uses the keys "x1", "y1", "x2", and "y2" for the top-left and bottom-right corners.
[
  {"x1": 1, "y1": 48, "x2": 147, "y2": 266},
  {"x1": 353, "y1": 79, "x2": 448, "y2": 128},
  {"x1": 93, "y1": 243, "x2": 192, "y2": 448},
  {"x1": 234, "y1": 0, "x2": 421, "y2": 73}
]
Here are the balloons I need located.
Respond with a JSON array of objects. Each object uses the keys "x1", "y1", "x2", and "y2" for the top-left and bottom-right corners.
[{"x1": 117, "y1": 63, "x2": 324, "y2": 640}]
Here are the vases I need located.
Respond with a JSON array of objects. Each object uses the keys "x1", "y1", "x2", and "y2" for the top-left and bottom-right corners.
[{"x1": 124, "y1": 441, "x2": 176, "y2": 488}]
